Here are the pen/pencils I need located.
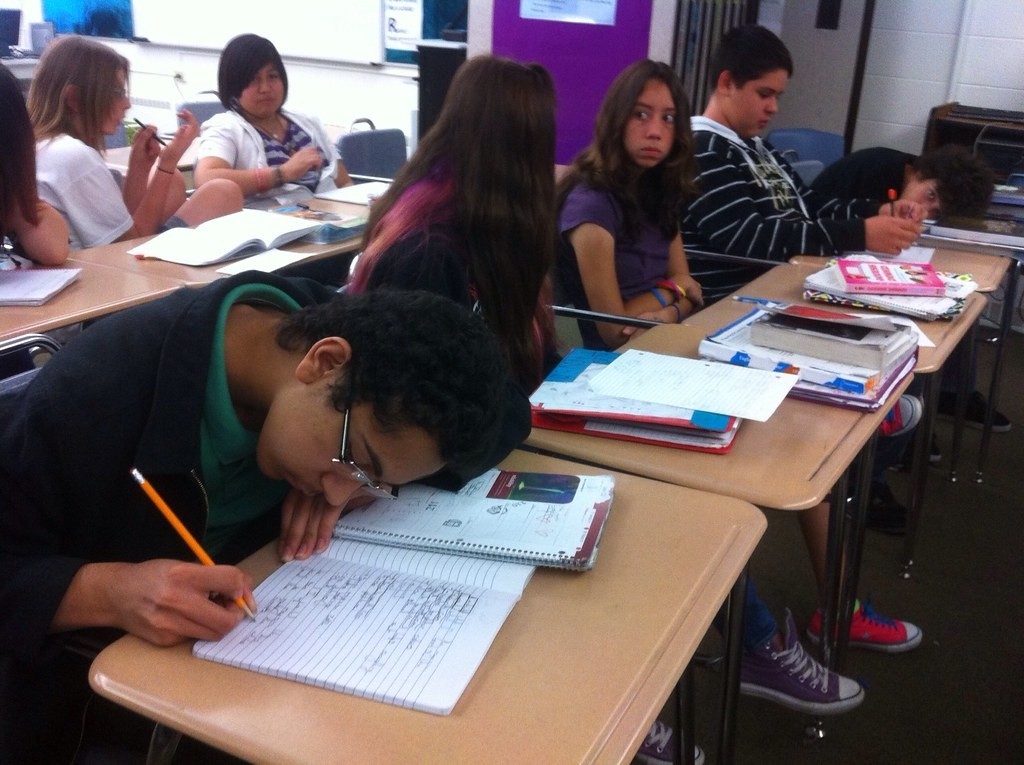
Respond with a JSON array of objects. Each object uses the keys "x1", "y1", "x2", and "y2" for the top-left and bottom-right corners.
[
  {"x1": 296, "y1": 202, "x2": 309, "y2": 210},
  {"x1": 731, "y1": 295, "x2": 782, "y2": 305},
  {"x1": 889, "y1": 189, "x2": 895, "y2": 217},
  {"x1": 133, "y1": 118, "x2": 167, "y2": 147},
  {"x1": 128, "y1": 467, "x2": 256, "y2": 624},
  {"x1": 312, "y1": 134, "x2": 319, "y2": 181}
]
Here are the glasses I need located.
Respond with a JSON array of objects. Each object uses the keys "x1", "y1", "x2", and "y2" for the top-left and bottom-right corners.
[
  {"x1": 110, "y1": 88, "x2": 127, "y2": 98},
  {"x1": 331, "y1": 371, "x2": 400, "y2": 502}
]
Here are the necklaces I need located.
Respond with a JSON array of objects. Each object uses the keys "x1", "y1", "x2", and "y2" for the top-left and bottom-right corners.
[{"x1": 257, "y1": 122, "x2": 278, "y2": 139}]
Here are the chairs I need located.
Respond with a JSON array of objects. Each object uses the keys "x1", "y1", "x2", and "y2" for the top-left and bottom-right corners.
[
  {"x1": 337, "y1": 118, "x2": 407, "y2": 184},
  {"x1": 543, "y1": 245, "x2": 784, "y2": 329},
  {"x1": 0, "y1": 333, "x2": 59, "y2": 396},
  {"x1": 768, "y1": 129, "x2": 846, "y2": 187},
  {"x1": 177, "y1": 91, "x2": 226, "y2": 137}
]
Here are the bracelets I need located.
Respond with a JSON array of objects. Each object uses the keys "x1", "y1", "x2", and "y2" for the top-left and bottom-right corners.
[
  {"x1": 651, "y1": 288, "x2": 666, "y2": 308},
  {"x1": 157, "y1": 166, "x2": 174, "y2": 174},
  {"x1": 891, "y1": 201, "x2": 894, "y2": 217},
  {"x1": 255, "y1": 165, "x2": 282, "y2": 192},
  {"x1": 672, "y1": 303, "x2": 681, "y2": 322},
  {"x1": 656, "y1": 279, "x2": 686, "y2": 300}
]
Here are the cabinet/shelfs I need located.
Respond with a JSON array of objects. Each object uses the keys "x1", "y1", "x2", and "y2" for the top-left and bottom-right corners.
[{"x1": 919, "y1": 102, "x2": 1024, "y2": 186}]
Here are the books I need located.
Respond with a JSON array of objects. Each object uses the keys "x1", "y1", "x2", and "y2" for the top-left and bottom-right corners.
[
  {"x1": 803, "y1": 254, "x2": 978, "y2": 320},
  {"x1": 0, "y1": 268, "x2": 82, "y2": 307},
  {"x1": 126, "y1": 210, "x2": 321, "y2": 266},
  {"x1": 333, "y1": 467, "x2": 615, "y2": 571},
  {"x1": 191, "y1": 537, "x2": 536, "y2": 716},
  {"x1": 698, "y1": 302, "x2": 919, "y2": 393}
]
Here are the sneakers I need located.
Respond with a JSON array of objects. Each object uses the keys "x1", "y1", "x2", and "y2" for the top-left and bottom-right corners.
[
  {"x1": 935, "y1": 391, "x2": 1012, "y2": 433},
  {"x1": 877, "y1": 395, "x2": 922, "y2": 437},
  {"x1": 738, "y1": 608, "x2": 865, "y2": 717},
  {"x1": 846, "y1": 482, "x2": 908, "y2": 533},
  {"x1": 911, "y1": 430, "x2": 941, "y2": 462},
  {"x1": 639, "y1": 722, "x2": 705, "y2": 765},
  {"x1": 807, "y1": 601, "x2": 922, "y2": 652}
]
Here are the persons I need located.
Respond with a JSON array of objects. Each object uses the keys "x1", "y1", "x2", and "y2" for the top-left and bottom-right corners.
[
  {"x1": 0, "y1": 269, "x2": 532, "y2": 765},
  {"x1": 23, "y1": 35, "x2": 243, "y2": 248},
  {"x1": 680, "y1": 24, "x2": 929, "y2": 533},
  {"x1": 193, "y1": 34, "x2": 372, "y2": 287},
  {"x1": 347, "y1": 56, "x2": 864, "y2": 765},
  {"x1": 556, "y1": 60, "x2": 923, "y2": 652},
  {"x1": 802, "y1": 144, "x2": 1009, "y2": 460},
  {"x1": 0, "y1": 64, "x2": 69, "y2": 266}
]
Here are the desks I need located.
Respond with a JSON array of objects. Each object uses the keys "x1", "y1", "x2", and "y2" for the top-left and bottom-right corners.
[
  {"x1": 0, "y1": 198, "x2": 370, "y2": 343},
  {"x1": 0, "y1": 58, "x2": 40, "y2": 80},
  {"x1": 99, "y1": 137, "x2": 194, "y2": 179},
  {"x1": 85, "y1": 215, "x2": 1024, "y2": 765}
]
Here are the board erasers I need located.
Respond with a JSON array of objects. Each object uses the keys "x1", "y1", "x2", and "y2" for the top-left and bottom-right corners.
[{"x1": 131, "y1": 36, "x2": 149, "y2": 42}]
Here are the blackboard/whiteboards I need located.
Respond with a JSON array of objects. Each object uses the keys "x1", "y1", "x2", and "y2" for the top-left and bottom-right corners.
[{"x1": 129, "y1": 0, "x2": 386, "y2": 68}]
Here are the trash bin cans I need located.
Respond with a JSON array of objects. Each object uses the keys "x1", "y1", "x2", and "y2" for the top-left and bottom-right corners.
[{"x1": 123, "y1": 121, "x2": 140, "y2": 147}]
